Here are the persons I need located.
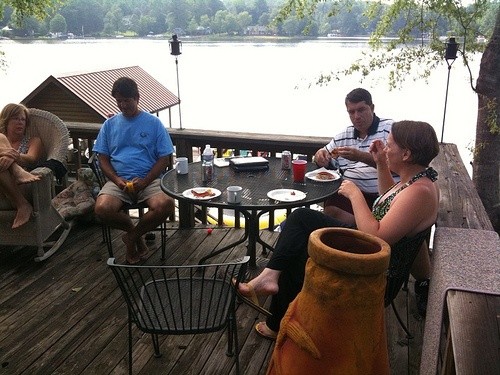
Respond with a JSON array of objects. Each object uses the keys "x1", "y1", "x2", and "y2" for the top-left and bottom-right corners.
[
  {"x1": 0, "y1": 103, "x2": 44, "y2": 229},
  {"x1": 315, "y1": 88, "x2": 401, "y2": 214},
  {"x1": 230, "y1": 121, "x2": 440, "y2": 339},
  {"x1": 92, "y1": 77, "x2": 174, "y2": 265}
]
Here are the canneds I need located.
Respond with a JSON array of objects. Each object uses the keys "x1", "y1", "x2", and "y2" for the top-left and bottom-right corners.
[
  {"x1": 281, "y1": 151, "x2": 291, "y2": 170},
  {"x1": 202, "y1": 161, "x2": 213, "y2": 182}
]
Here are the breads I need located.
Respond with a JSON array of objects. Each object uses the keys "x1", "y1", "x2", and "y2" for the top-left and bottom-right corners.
[{"x1": 317, "y1": 172, "x2": 335, "y2": 180}]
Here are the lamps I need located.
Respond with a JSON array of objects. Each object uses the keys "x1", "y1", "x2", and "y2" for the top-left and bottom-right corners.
[
  {"x1": 168, "y1": 35, "x2": 186, "y2": 131},
  {"x1": 441, "y1": 38, "x2": 460, "y2": 144}
]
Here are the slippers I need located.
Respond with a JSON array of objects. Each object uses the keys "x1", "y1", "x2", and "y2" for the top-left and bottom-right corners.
[
  {"x1": 231, "y1": 278, "x2": 272, "y2": 316},
  {"x1": 256, "y1": 322, "x2": 278, "y2": 339}
]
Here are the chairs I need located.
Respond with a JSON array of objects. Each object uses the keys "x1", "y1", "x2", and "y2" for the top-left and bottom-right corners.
[
  {"x1": 0, "y1": 107, "x2": 76, "y2": 263},
  {"x1": 107, "y1": 256, "x2": 250, "y2": 375},
  {"x1": 87, "y1": 140, "x2": 170, "y2": 264},
  {"x1": 384, "y1": 229, "x2": 432, "y2": 338}
]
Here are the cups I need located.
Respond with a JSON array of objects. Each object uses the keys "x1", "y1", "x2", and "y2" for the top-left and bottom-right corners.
[
  {"x1": 175, "y1": 157, "x2": 188, "y2": 174},
  {"x1": 227, "y1": 185, "x2": 242, "y2": 203},
  {"x1": 292, "y1": 159, "x2": 307, "y2": 183}
]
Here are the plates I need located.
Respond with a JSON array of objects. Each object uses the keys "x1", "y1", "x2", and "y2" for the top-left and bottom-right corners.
[
  {"x1": 267, "y1": 189, "x2": 306, "y2": 202},
  {"x1": 305, "y1": 171, "x2": 341, "y2": 182},
  {"x1": 182, "y1": 187, "x2": 221, "y2": 199}
]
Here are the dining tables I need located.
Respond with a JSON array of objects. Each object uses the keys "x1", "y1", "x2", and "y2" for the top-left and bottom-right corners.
[{"x1": 160, "y1": 156, "x2": 343, "y2": 282}]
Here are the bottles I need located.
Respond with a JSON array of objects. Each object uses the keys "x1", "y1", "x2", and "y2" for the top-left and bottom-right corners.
[
  {"x1": 202, "y1": 163, "x2": 213, "y2": 182},
  {"x1": 202, "y1": 145, "x2": 214, "y2": 174},
  {"x1": 282, "y1": 151, "x2": 291, "y2": 170}
]
